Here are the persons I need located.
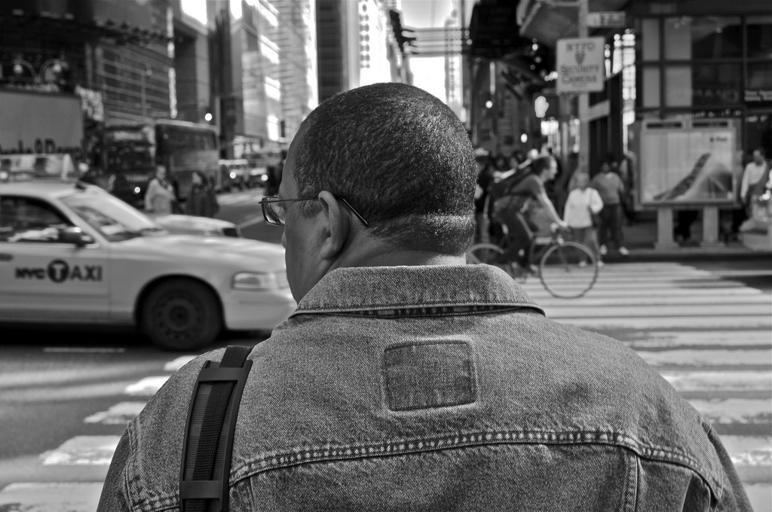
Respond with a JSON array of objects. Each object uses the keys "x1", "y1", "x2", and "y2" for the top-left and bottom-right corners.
[
  {"x1": 263, "y1": 163, "x2": 282, "y2": 195},
  {"x1": 740, "y1": 147, "x2": 772, "y2": 235},
  {"x1": 476, "y1": 142, "x2": 629, "y2": 275},
  {"x1": 144, "y1": 163, "x2": 177, "y2": 215},
  {"x1": 95, "y1": 82, "x2": 755, "y2": 512},
  {"x1": 186, "y1": 170, "x2": 220, "y2": 217}
]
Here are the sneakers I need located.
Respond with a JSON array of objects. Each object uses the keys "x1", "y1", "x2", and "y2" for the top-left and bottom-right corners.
[{"x1": 578, "y1": 244, "x2": 629, "y2": 268}]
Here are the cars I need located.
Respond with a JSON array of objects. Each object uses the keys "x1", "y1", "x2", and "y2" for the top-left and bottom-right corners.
[
  {"x1": 87, "y1": 120, "x2": 286, "y2": 203},
  {"x1": 2, "y1": 180, "x2": 297, "y2": 351}
]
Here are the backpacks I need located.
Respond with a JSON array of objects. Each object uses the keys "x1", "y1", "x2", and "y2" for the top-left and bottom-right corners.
[{"x1": 491, "y1": 164, "x2": 538, "y2": 198}]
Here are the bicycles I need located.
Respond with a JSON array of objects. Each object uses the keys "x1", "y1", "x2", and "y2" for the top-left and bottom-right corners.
[{"x1": 461, "y1": 221, "x2": 599, "y2": 300}]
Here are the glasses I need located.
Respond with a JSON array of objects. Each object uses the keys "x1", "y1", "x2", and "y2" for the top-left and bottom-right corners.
[{"x1": 257, "y1": 194, "x2": 370, "y2": 228}]
